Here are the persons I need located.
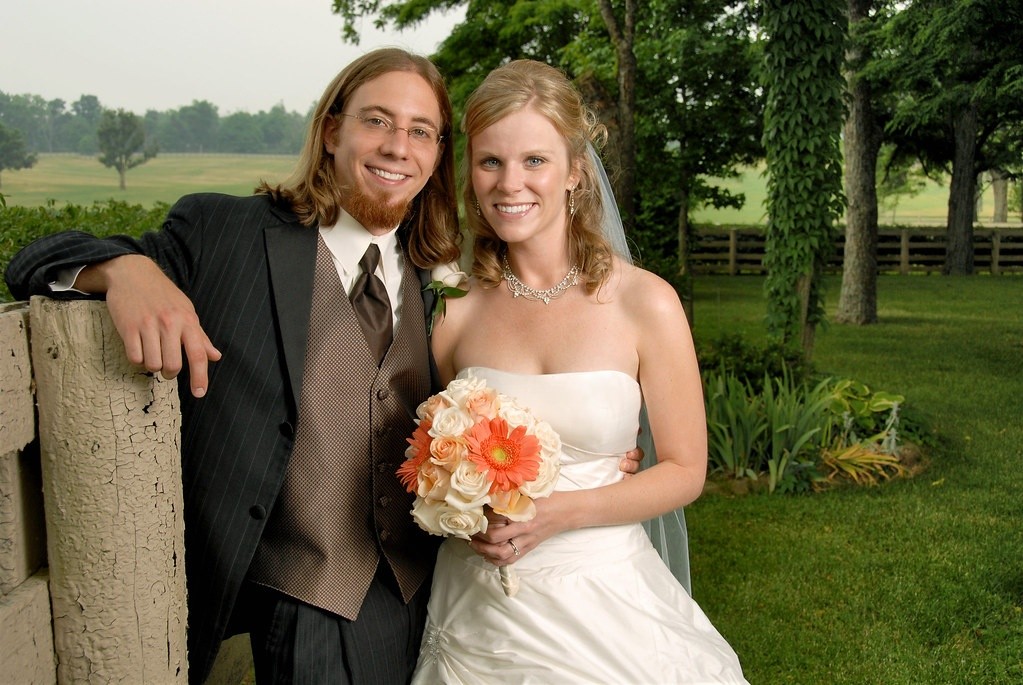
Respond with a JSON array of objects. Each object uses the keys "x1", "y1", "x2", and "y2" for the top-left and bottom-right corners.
[{"x1": 4, "y1": 47, "x2": 708, "y2": 685}]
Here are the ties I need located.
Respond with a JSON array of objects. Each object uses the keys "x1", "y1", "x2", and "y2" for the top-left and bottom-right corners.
[{"x1": 349, "y1": 243, "x2": 394, "y2": 367}]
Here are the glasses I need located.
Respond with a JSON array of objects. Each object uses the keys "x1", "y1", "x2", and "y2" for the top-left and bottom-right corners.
[{"x1": 336, "y1": 111, "x2": 444, "y2": 148}]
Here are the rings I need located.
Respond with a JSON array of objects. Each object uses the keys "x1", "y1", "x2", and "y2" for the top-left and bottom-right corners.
[{"x1": 510, "y1": 540, "x2": 521, "y2": 556}]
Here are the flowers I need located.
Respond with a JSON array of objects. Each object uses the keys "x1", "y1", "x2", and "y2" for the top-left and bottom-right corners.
[
  {"x1": 425, "y1": 260, "x2": 472, "y2": 339},
  {"x1": 394, "y1": 369, "x2": 561, "y2": 595}
]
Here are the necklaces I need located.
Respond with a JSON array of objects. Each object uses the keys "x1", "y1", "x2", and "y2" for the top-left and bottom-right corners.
[{"x1": 502, "y1": 255, "x2": 582, "y2": 305}]
